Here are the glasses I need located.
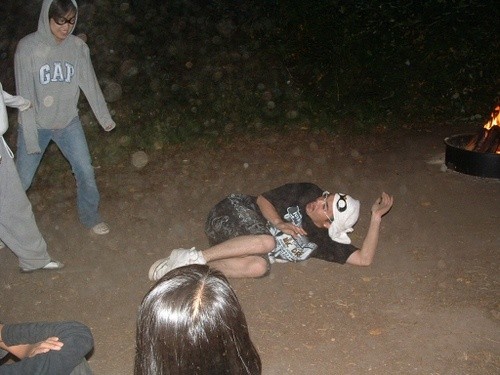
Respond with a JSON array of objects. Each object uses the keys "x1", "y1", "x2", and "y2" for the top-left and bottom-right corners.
[
  {"x1": 52, "y1": 14, "x2": 76, "y2": 25},
  {"x1": 322, "y1": 190, "x2": 332, "y2": 222}
]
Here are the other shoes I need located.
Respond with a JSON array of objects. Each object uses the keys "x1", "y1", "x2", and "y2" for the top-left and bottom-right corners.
[
  {"x1": 80, "y1": 217, "x2": 110, "y2": 235},
  {"x1": 20, "y1": 257, "x2": 64, "y2": 273}
]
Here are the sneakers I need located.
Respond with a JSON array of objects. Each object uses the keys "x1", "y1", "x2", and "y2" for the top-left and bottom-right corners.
[{"x1": 148, "y1": 246, "x2": 199, "y2": 282}]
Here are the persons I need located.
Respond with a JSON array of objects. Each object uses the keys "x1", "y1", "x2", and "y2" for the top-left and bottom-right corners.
[
  {"x1": 147, "y1": 182, "x2": 393, "y2": 281},
  {"x1": 0, "y1": 0, "x2": 116, "y2": 250},
  {"x1": 0, "y1": 322, "x2": 94, "y2": 375},
  {"x1": 0, "y1": 82, "x2": 63, "y2": 271},
  {"x1": 134, "y1": 264, "x2": 262, "y2": 375}
]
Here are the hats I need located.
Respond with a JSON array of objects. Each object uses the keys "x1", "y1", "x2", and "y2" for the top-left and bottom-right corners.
[{"x1": 328, "y1": 192, "x2": 361, "y2": 244}]
